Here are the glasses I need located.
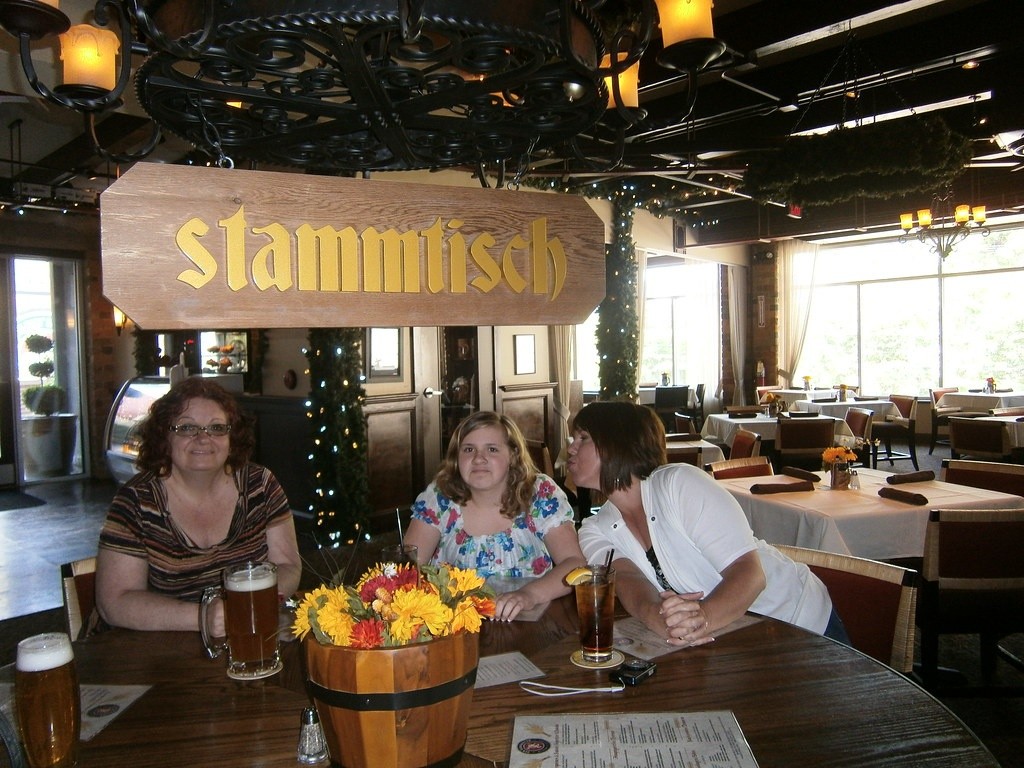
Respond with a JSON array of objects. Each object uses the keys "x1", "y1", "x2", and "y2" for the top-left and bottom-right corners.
[{"x1": 165, "y1": 423, "x2": 231, "y2": 437}]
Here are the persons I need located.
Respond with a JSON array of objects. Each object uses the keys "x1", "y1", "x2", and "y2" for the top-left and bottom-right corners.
[
  {"x1": 565, "y1": 401, "x2": 853, "y2": 648},
  {"x1": 402, "y1": 410, "x2": 588, "y2": 622},
  {"x1": 93, "y1": 376, "x2": 302, "y2": 639}
]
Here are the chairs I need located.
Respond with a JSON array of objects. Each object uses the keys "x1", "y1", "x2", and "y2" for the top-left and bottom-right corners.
[{"x1": 0, "y1": 371, "x2": 1024, "y2": 768}]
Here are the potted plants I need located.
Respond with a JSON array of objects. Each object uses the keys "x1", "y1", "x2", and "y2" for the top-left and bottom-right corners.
[{"x1": 21, "y1": 335, "x2": 79, "y2": 479}]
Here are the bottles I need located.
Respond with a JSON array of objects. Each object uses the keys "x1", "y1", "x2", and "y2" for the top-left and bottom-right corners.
[
  {"x1": 985, "y1": 386, "x2": 991, "y2": 394},
  {"x1": 296, "y1": 706, "x2": 328, "y2": 766},
  {"x1": 765, "y1": 408, "x2": 770, "y2": 417},
  {"x1": 847, "y1": 470, "x2": 860, "y2": 490}
]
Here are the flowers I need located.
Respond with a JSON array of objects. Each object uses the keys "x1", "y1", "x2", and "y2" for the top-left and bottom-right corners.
[
  {"x1": 802, "y1": 375, "x2": 813, "y2": 381},
  {"x1": 289, "y1": 554, "x2": 500, "y2": 649},
  {"x1": 766, "y1": 392, "x2": 781, "y2": 403},
  {"x1": 986, "y1": 376, "x2": 995, "y2": 382},
  {"x1": 821, "y1": 446, "x2": 858, "y2": 472}
]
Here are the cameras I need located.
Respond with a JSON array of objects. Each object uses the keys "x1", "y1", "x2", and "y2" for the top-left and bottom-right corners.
[{"x1": 609, "y1": 658, "x2": 657, "y2": 687}]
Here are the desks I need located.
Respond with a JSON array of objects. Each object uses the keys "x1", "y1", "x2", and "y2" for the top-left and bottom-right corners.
[
  {"x1": 794, "y1": 398, "x2": 893, "y2": 461},
  {"x1": 638, "y1": 384, "x2": 689, "y2": 414},
  {"x1": 943, "y1": 386, "x2": 1024, "y2": 409},
  {"x1": 769, "y1": 388, "x2": 853, "y2": 403},
  {"x1": 708, "y1": 411, "x2": 847, "y2": 445},
  {"x1": 0, "y1": 545, "x2": 1000, "y2": 768},
  {"x1": 973, "y1": 414, "x2": 1024, "y2": 448},
  {"x1": 709, "y1": 465, "x2": 1024, "y2": 567},
  {"x1": 666, "y1": 432, "x2": 720, "y2": 464}
]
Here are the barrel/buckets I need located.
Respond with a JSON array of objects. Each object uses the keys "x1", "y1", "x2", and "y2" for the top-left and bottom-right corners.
[{"x1": 298, "y1": 628, "x2": 479, "y2": 768}]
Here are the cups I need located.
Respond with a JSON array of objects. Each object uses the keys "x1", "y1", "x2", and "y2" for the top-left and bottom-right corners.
[
  {"x1": 575, "y1": 564, "x2": 616, "y2": 663},
  {"x1": 199, "y1": 562, "x2": 280, "y2": 679},
  {"x1": 381, "y1": 544, "x2": 418, "y2": 571},
  {"x1": 458, "y1": 339, "x2": 470, "y2": 361},
  {"x1": 14, "y1": 632, "x2": 79, "y2": 768}
]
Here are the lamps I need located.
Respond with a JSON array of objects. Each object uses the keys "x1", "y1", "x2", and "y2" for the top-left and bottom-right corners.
[{"x1": 0, "y1": 0, "x2": 995, "y2": 339}]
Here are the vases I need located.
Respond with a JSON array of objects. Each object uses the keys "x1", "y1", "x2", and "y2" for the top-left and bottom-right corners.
[
  {"x1": 830, "y1": 464, "x2": 849, "y2": 490},
  {"x1": 804, "y1": 381, "x2": 811, "y2": 390},
  {"x1": 988, "y1": 382, "x2": 993, "y2": 392},
  {"x1": 770, "y1": 403, "x2": 780, "y2": 417}
]
